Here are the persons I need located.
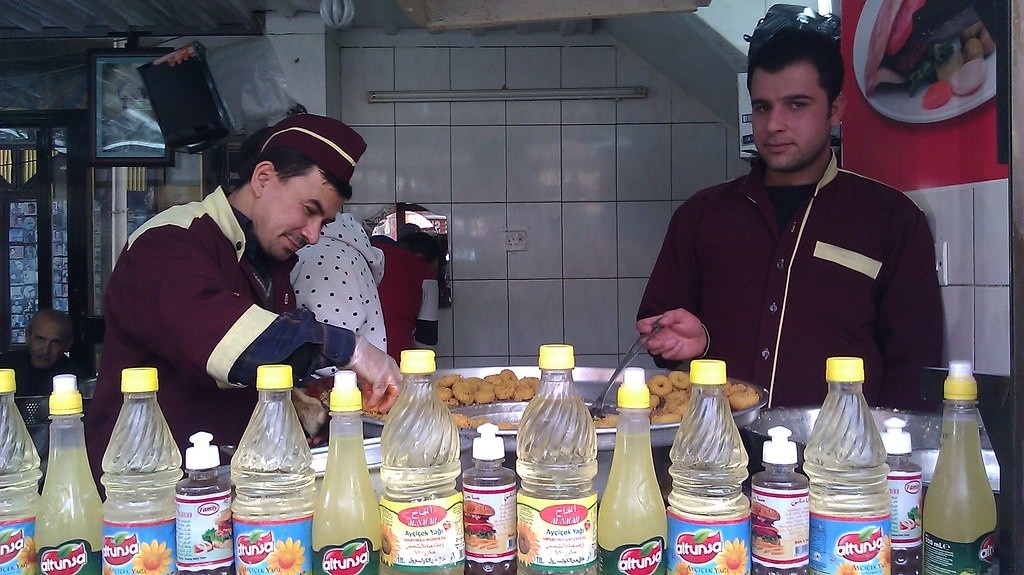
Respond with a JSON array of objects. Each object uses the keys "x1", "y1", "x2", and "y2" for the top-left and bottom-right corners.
[
  {"x1": 83, "y1": 113, "x2": 404, "y2": 503},
  {"x1": 370, "y1": 232, "x2": 439, "y2": 365},
  {"x1": 637, "y1": 29, "x2": 946, "y2": 412},
  {"x1": 0, "y1": 308, "x2": 81, "y2": 397},
  {"x1": 290, "y1": 212, "x2": 387, "y2": 354}
]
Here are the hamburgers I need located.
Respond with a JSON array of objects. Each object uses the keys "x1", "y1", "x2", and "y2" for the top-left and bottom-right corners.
[
  {"x1": 751, "y1": 502, "x2": 781, "y2": 544},
  {"x1": 210, "y1": 508, "x2": 233, "y2": 548},
  {"x1": 463, "y1": 501, "x2": 498, "y2": 540}
]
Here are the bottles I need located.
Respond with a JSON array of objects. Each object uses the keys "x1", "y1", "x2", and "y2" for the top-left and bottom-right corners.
[
  {"x1": 33, "y1": 374, "x2": 104, "y2": 575},
  {"x1": 229, "y1": 365, "x2": 317, "y2": 575},
  {"x1": 925, "y1": 360, "x2": 999, "y2": 575},
  {"x1": 751, "y1": 426, "x2": 811, "y2": 575},
  {"x1": 311, "y1": 370, "x2": 382, "y2": 575},
  {"x1": 379, "y1": 349, "x2": 465, "y2": 575},
  {"x1": 598, "y1": 366, "x2": 666, "y2": 575},
  {"x1": 175, "y1": 431, "x2": 234, "y2": 575},
  {"x1": 461, "y1": 423, "x2": 517, "y2": 575},
  {"x1": 881, "y1": 417, "x2": 924, "y2": 575},
  {"x1": 1, "y1": 370, "x2": 46, "y2": 575},
  {"x1": 802, "y1": 357, "x2": 893, "y2": 575},
  {"x1": 666, "y1": 359, "x2": 752, "y2": 575},
  {"x1": 516, "y1": 344, "x2": 599, "y2": 575},
  {"x1": 101, "y1": 367, "x2": 184, "y2": 575}
]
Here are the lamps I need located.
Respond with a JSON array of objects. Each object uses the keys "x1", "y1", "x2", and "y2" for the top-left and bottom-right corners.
[{"x1": 367, "y1": 83, "x2": 647, "y2": 102}]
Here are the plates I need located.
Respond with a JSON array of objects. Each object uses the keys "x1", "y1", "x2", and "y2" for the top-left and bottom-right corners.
[{"x1": 852, "y1": 0, "x2": 996, "y2": 123}]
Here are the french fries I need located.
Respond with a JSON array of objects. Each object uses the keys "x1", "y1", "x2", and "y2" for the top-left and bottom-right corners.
[
  {"x1": 752, "y1": 539, "x2": 786, "y2": 555},
  {"x1": 464, "y1": 536, "x2": 499, "y2": 550}
]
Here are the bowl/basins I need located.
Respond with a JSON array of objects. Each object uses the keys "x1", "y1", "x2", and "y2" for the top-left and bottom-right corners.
[
  {"x1": 744, "y1": 404, "x2": 995, "y2": 452},
  {"x1": 911, "y1": 435, "x2": 1000, "y2": 502}
]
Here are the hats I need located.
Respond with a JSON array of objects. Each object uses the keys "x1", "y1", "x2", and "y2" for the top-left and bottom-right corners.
[{"x1": 258, "y1": 114, "x2": 369, "y2": 182}]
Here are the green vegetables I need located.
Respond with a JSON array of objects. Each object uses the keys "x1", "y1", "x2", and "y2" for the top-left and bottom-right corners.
[{"x1": 202, "y1": 528, "x2": 216, "y2": 543}]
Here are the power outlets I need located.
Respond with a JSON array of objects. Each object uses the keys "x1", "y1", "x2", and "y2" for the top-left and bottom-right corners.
[{"x1": 502, "y1": 230, "x2": 526, "y2": 251}]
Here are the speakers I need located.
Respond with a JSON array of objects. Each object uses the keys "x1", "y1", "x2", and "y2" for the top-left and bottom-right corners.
[{"x1": 135, "y1": 40, "x2": 234, "y2": 155}]
